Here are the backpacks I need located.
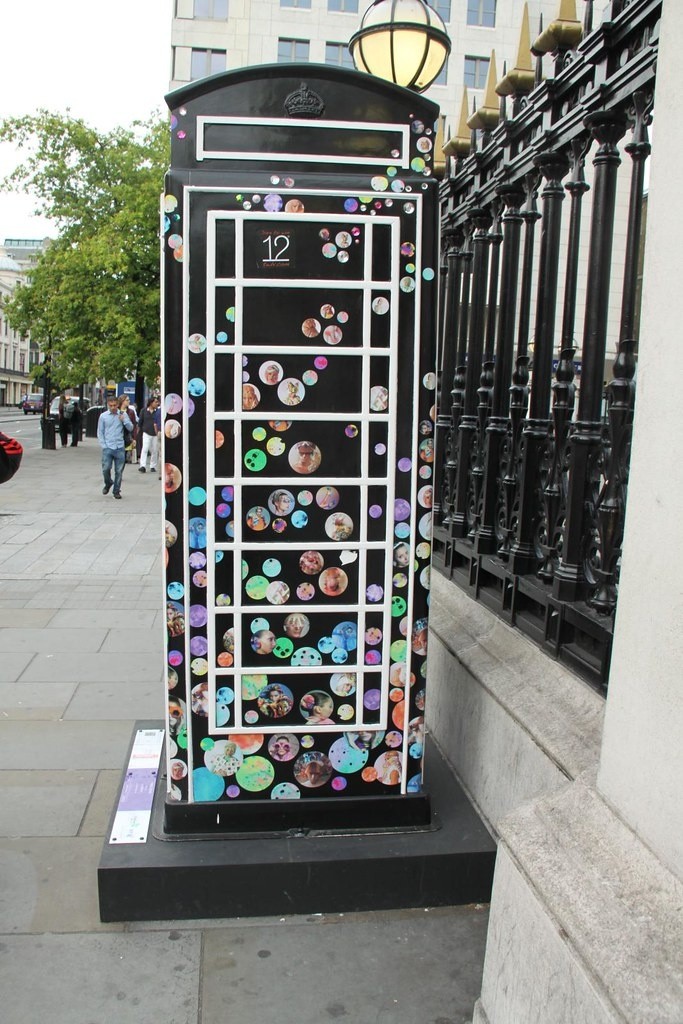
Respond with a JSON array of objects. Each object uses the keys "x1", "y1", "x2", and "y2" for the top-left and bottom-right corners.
[{"x1": 62, "y1": 402, "x2": 75, "y2": 419}]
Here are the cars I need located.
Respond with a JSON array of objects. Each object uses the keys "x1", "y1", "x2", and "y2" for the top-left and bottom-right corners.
[{"x1": 22, "y1": 394, "x2": 43, "y2": 415}]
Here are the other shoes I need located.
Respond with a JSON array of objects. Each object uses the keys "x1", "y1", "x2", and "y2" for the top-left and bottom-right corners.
[
  {"x1": 102, "y1": 484, "x2": 112, "y2": 494},
  {"x1": 138, "y1": 467, "x2": 146, "y2": 473},
  {"x1": 114, "y1": 492, "x2": 121, "y2": 499},
  {"x1": 151, "y1": 468, "x2": 155, "y2": 472}
]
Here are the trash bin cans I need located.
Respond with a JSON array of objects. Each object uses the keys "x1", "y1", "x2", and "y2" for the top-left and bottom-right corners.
[{"x1": 86, "y1": 406, "x2": 106, "y2": 437}]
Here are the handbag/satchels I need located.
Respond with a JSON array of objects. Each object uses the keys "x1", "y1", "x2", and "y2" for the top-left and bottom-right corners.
[{"x1": 122, "y1": 424, "x2": 131, "y2": 448}]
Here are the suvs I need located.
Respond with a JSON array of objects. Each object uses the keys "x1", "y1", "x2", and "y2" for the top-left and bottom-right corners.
[{"x1": 49, "y1": 395, "x2": 91, "y2": 429}]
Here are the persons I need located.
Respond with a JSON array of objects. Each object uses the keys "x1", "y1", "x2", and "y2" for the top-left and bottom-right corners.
[
  {"x1": 55, "y1": 393, "x2": 160, "y2": 472},
  {"x1": 96, "y1": 396, "x2": 134, "y2": 499}
]
